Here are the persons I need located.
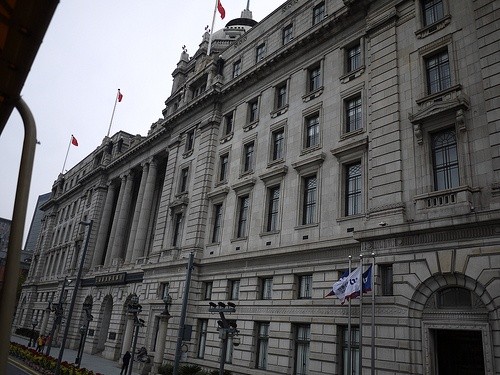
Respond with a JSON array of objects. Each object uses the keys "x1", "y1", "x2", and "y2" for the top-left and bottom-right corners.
[
  {"x1": 35, "y1": 334, "x2": 50, "y2": 355},
  {"x1": 120, "y1": 351, "x2": 131, "y2": 375}
]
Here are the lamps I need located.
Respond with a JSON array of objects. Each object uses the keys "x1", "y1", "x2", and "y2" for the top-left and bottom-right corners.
[{"x1": 24, "y1": 257, "x2": 32, "y2": 262}]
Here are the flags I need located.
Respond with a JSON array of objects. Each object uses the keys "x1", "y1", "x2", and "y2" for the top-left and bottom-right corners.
[
  {"x1": 70, "y1": 134, "x2": 78, "y2": 146},
  {"x1": 325, "y1": 262, "x2": 372, "y2": 300},
  {"x1": 332, "y1": 254, "x2": 361, "y2": 304},
  {"x1": 216, "y1": 0, "x2": 226, "y2": 20},
  {"x1": 117, "y1": 89, "x2": 122, "y2": 102}
]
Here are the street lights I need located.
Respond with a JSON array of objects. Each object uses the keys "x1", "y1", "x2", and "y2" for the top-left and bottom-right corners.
[
  {"x1": 54, "y1": 219, "x2": 93, "y2": 375},
  {"x1": 75, "y1": 324, "x2": 87, "y2": 364}
]
